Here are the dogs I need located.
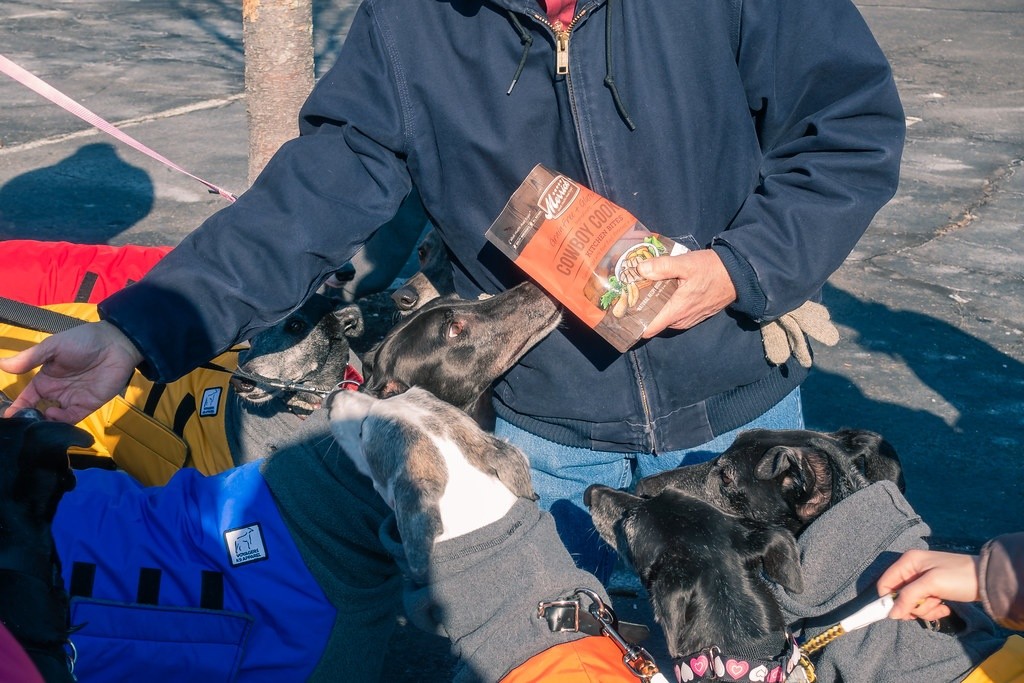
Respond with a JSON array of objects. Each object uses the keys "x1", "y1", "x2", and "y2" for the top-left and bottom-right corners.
[{"x1": 1, "y1": 239, "x2": 1024, "y2": 683}]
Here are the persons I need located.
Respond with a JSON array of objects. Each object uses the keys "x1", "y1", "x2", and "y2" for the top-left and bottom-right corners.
[
  {"x1": 876, "y1": 531, "x2": 1023, "y2": 637},
  {"x1": 2, "y1": 1, "x2": 906, "y2": 683}
]
